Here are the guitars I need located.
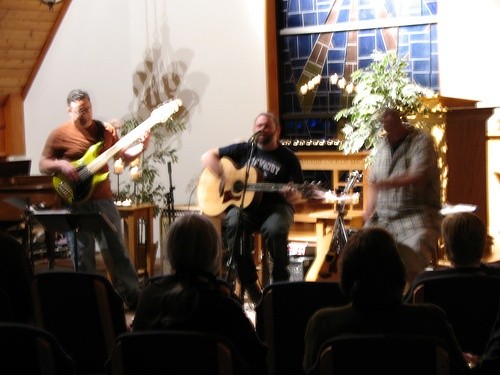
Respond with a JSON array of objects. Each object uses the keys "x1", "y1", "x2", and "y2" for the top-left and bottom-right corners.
[
  {"x1": 52, "y1": 98, "x2": 182, "y2": 210},
  {"x1": 195, "y1": 154, "x2": 321, "y2": 218},
  {"x1": 305, "y1": 170, "x2": 362, "y2": 283}
]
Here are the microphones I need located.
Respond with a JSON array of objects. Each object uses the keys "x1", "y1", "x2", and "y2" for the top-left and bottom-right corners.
[{"x1": 251, "y1": 129, "x2": 266, "y2": 137}]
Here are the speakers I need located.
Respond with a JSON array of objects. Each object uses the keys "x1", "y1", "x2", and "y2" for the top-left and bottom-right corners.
[{"x1": 160, "y1": 203, "x2": 202, "y2": 256}]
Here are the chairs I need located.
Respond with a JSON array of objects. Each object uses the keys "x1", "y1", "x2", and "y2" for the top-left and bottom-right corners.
[{"x1": 0, "y1": 236, "x2": 500, "y2": 375}]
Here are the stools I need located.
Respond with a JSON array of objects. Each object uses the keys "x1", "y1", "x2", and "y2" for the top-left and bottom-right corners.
[
  {"x1": 114, "y1": 204, "x2": 158, "y2": 278},
  {"x1": 215, "y1": 218, "x2": 270, "y2": 295}
]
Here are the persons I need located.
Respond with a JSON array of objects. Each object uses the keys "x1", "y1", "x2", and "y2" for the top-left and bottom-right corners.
[
  {"x1": 404, "y1": 214, "x2": 500, "y2": 304},
  {"x1": 460, "y1": 306, "x2": 500, "y2": 375},
  {"x1": 39, "y1": 89, "x2": 151, "y2": 315},
  {"x1": 361, "y1": 109, "x2": 444, "y2": 286},
  {"x1": 304, "y1": 229, "x2": 468, "y2": 375},
  {"x1": 131, "y1": 217, "x2": 273, "y2": 375},
  {"x1": 0, "y1": 234, "x2": 55, "y2": 374},
  {"x1": 200, "y1": 114, "x2": 310, "y2": 309}
]
